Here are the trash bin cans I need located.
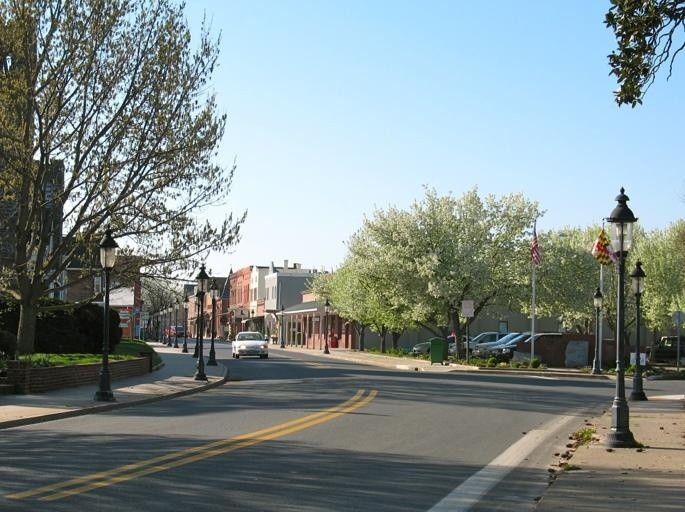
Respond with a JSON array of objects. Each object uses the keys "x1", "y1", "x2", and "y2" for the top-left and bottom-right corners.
[{"x1": 431, "y1": 337, "x2": 448, "y2": 365}]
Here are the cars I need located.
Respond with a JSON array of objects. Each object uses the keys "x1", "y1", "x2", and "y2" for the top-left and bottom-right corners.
[
  {"x1": 409, "y1": 328, "x2": 684, "y2": 377},
  {"x1": 229, "y1": 330, "x2": 270, "y2": 359}
]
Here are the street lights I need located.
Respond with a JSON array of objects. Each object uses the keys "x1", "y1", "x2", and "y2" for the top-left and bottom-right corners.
[
  {"x1": 206, "y1": 278, "x2": 219, "y2": 366},
  {"x1": 590, "y1": 286, "x2": 605, "y2": 375},
  {"x1": 628, "y1": 256, "x2": 651, "y2": 402},
  {"x1": 193, "y1": 263, "x2": 209, "y2": 380},
  {"x1": 322, "y1": 298, "x2": 331, "y2": 354},
  {"x1": 600, "y1": 186, "x2": 647, "y2": 450},
  {"x1": 280, "y1": 304, "x2": 286, "y2": 348},
  {"x1": 92, "y1": 223, "x2": 118, "y2": 403},
  {"x1": 147, "y1": 293, "x2": 190, "y2": 354},
  {"x1": 192, "y1": 288, "x2": 201, "y2": 358},
  {"x1": 251, "y1": 308, "x2": 255, "y2": 331}
]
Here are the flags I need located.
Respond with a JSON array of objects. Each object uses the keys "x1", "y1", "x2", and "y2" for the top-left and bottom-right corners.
[
  {"x1": 530, "y1": 224, "x2": 541, "y2": 266},
  {"x1": 591, "y1": 227, "x2": 613, "y2": 266}
]
